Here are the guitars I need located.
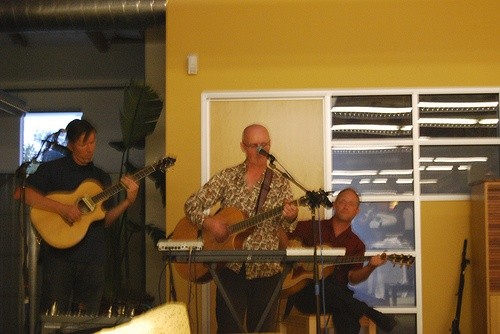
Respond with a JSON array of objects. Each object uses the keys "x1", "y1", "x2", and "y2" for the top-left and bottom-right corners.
[
  {"x1": 28, "y1": 157, "x2": 176, "y2": 253},
  {"x1": 281, "y1": 239, "x2": 415, "y2": 297},
  {"x1": 171, "y1": 189, "x2": 307, "y2": 283}
]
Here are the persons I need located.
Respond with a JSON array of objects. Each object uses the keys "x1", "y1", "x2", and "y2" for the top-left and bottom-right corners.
[
  {"x1": 14, "y1": 120, "x2": 139, "y2": 315},
  {"x1": 288, "y1": 187, "x2": 398, "y2": 334},
  {"x1": 378, "y1": 234, "x2": 410, "y2": 307},
  {"x1": 185, "y1": 124, "x2": 299, "y2": 334}
]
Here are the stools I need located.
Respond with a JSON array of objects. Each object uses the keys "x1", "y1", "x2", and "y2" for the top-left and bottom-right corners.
[{"x1": 274, "y1": 299, "x2": 376, "y2": 334}]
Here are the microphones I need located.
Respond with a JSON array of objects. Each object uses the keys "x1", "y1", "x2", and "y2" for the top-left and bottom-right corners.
[
  {"x1": 47, "y1": 129, "x2": 65, "y2": 138},
  {"x1": 256, "y1": 146, "x2": 277, "y2": 162}
]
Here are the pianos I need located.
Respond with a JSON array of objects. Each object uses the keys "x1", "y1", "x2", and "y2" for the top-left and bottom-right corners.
[{"x1": 157, "y1": 241, "x2": 347, "y2": 334}]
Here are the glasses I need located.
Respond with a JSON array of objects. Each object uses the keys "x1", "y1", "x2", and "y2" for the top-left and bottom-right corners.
[{"x1": 243, "y1": 140, "x2": 271, "y2": 150}]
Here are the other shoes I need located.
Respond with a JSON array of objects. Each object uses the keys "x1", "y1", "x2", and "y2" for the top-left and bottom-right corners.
[{"x1": 367, "y1": 308, "x2": 398, "y2": 332}]
